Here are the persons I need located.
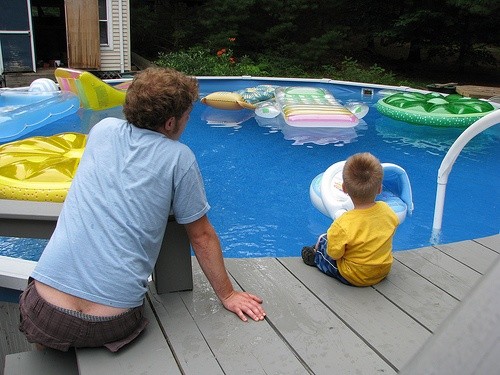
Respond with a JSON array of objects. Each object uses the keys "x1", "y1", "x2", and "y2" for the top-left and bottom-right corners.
[
  {"x1": 16, "y1": 64, "x2": 268, "y2": 357},
  {"x1": 300, "y1": 152, "x2": 401, "y2": 288}
]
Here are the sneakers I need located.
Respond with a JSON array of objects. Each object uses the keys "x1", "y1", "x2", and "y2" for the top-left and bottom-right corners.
[{"x1": 302, "y1": 245, "x2": 316, "y2": 266}]
